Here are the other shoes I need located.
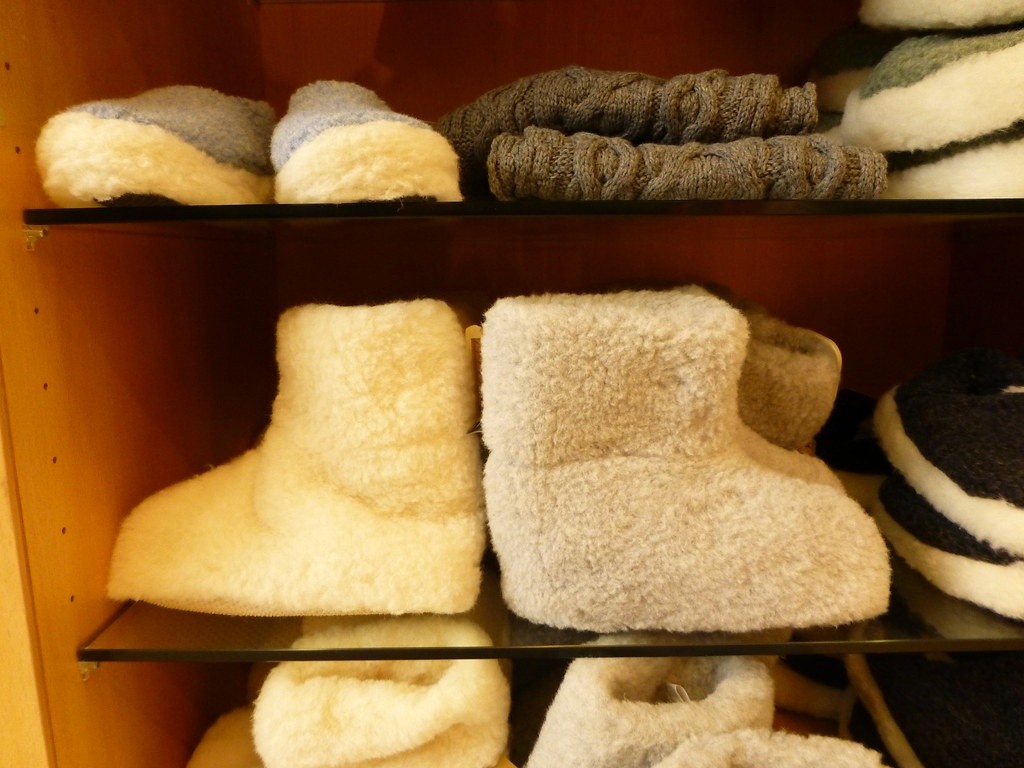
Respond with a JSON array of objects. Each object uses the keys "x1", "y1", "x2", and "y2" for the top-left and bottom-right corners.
[
  {"x1": 869, "y1": 369, "x2": 1024, "y2": 626},
  {"x1": 35, "y1": 86, "x2": 273, "y2": 206},
  {"x1": 837, "y1": 619, "x2": 1024, "y2": 768},
  {"x1": 267, "y1": 79, "x2": 466, "y2": 203}
]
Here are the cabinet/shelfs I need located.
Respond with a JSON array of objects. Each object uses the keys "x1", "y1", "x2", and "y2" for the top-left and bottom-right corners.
[{"x1": 22, "y1": 198, "x2": 1023, "y2": 680}]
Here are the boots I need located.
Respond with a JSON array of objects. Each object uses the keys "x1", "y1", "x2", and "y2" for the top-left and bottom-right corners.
[
  {"x1": 183, "y1": 615, "x2": 889, "y2": 768},
  {"x1": 481, "y1": 282, "x2": 893, "y2": 632},
  {"x1": 107, "y1": 298, "x2": 488, "y2": 616}
]
